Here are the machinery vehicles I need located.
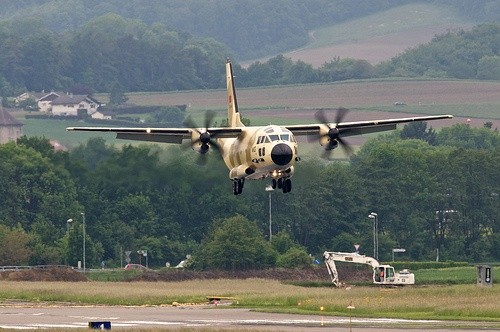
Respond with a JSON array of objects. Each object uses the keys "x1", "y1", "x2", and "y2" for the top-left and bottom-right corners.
[{"x1": 323, "y1": 250, "x2": 416, "y2": 290}]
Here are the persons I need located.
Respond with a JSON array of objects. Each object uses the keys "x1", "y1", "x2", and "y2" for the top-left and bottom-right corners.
[{"x1": 379, "y1": 270, "x2": 384, "y2": 276}]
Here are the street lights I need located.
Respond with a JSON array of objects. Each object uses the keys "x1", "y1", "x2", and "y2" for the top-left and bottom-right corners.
[
  {"x1": 67, "y1": 218, "x2": 73, "y2": 231},
  {"x1": 80, "y1": 212, "x2": 85, "y2": 272},
  {"x1": 370, "y1": 212, "x2": 379, "y2": 261},
  {"x1": 368, "y1": 214, "x2": 375, "y2": 259}
]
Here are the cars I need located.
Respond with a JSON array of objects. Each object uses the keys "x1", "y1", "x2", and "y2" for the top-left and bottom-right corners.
[{"x1": 123, "y1": 263, "x2": 153, "y2": 273}]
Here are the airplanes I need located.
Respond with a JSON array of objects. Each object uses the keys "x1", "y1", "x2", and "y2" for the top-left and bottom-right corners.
[{"x1": 65, "y1": 56, "x2": 454, "y2": 196}]
